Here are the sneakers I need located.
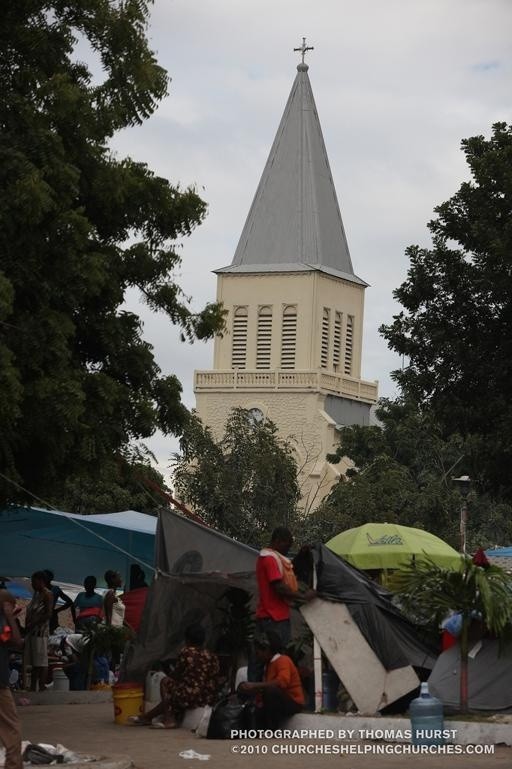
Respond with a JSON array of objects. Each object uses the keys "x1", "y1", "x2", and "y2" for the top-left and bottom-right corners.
[{"x1": 128, "y1": 715, "x2": 179, "y2": 730}]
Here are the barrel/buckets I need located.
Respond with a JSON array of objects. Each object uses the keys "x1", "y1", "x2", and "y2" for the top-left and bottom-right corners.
[
  {"x1": 111, "y1": 683, "x2": 146, "y2": 725},
  {"x1": 52, "y1": 669, "x2": 70, "y2": 690},
  {"x1": 409, "y1": 680, "x2": 444, "y2": 745}
]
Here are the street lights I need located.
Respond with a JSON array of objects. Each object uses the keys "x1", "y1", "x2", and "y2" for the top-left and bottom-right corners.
[{"x1": 454, "y1": 476, "x2": 471, "y2": 555}]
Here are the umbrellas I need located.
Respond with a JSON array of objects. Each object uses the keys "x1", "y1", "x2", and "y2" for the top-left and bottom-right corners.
[{"x1": 324, "y1": 522, "x2": 463, "y2": 589}]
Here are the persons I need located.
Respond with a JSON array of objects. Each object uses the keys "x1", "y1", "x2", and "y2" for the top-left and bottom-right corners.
[
  {"x1": 127, "y1": 624, "x2": 221, "y2": 729},
  {"x1": 237, "y1": 632, "x2": 305, "y2": 737},
  {"x1": 255, "y1": 525, "x2": 316, "y2": 664},
  {"x1": 0, "y1": 569, "x2": 149, "y2": 769}
]
